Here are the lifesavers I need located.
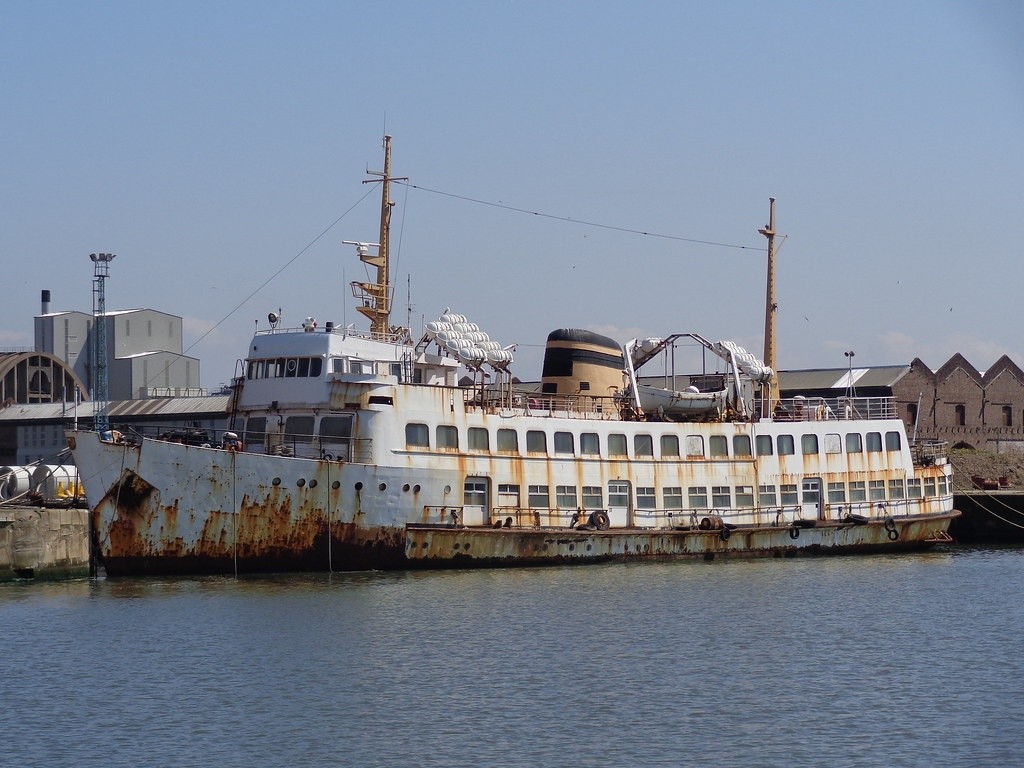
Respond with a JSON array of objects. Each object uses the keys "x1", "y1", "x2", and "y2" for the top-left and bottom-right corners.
[
  {"x1": 719, "y1": 528, "x2": 731, "y2": 540},
  {"x1": 884, "y1": 518, "x2": 896, "y2": 532},
  {"x1": 888, "y1": 529, "x2": 899, "y2": 541},
  {"x1": 790, "y1": 527, "x2": 800, "y2": 539},
  {"x1": 287, "y1": 359, "x2": 297, "y2": 373}
]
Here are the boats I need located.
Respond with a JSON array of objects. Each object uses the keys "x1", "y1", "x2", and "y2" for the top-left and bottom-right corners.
[
  {"x1": 62, "y1": 135, "x2": 963, "y2": 569},
  {"x1": 627, "y1": 381, "x2": 730, "y2": 419}
]
